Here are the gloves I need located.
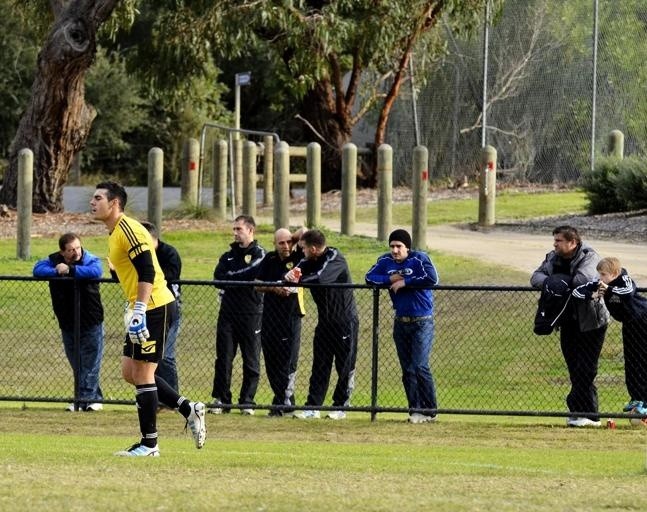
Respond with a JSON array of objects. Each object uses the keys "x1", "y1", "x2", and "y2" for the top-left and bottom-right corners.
[{"x1": 124, "y1": 302, "x2": 151, "y2": 345}]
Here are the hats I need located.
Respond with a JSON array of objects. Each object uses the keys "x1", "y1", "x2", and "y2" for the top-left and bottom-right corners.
[{"x1": 388, "y1": 229, "x2": 411, "y2": 250}]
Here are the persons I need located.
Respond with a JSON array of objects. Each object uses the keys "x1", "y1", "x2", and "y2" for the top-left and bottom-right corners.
[
  {"x1": 571, "y1": 258, "x2": 647, "y2": 416},
  {"x1": 208, "y1": 215, "x2": 267, "y2": 414},
  {"x1": 33, "y1": 232, "x2": 105, "y2": 412},
  {"x1": 365, "y1": 229, "x2": 439, "y2": 422},
  {"x1": 89, "y1": 183, "x2": 208, "y2": 458},
  {"x1": 284, "y1": 229, "x2": 359, "y2": 419},
  {"x1": 105, "y1": 221, "x2": 181, "y2": 412},
  {"x1": 529, "y1": 225, "x2": 605, "y2": 429},
  {"x1": 253, "y1": 228, "x2": 307, "y2": 417}
]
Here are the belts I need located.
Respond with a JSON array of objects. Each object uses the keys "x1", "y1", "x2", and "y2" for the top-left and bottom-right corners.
[{"x1": 393, "y1": 314, "x2": 434, "y2": 323}]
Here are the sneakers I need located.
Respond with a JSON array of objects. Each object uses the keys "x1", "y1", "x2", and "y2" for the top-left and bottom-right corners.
[
  {"x1": 407, "y1": 412, "x2": 438, "y2": 424},
  {"x1": 207, "y1": 399, "x2": 225, "y2": 415},
  {"x1": 85, "y1": 402, "x2": 103, "y2": 411},
  {"x1": 623, "y1": 398, "x2": 647, "y2": 417},
  {"x1": 292, "y1": 408, "x2": 321, "y2": 420},
  {"x1": 184, "y1": 400, "x2": 207, "y2": 448},
  {"x1": 113, "y1": 443, "x2": 160, "y2": 459},
  {"x1": 239, "y1": 408, "x2": 255, "y2": 415},
  {"x1": 65, "y1": 401, "x2": 83, "y2": 411},
  {"x1": 325, "y1": 411, "x2": 345, "y2": 420},
  {"x1": 568, "y1": 417, "x2": 601, "y2": 428}
]
